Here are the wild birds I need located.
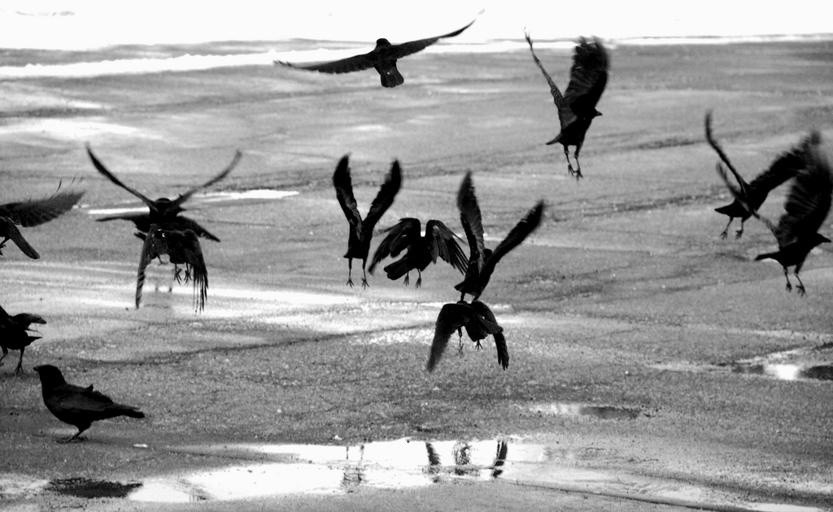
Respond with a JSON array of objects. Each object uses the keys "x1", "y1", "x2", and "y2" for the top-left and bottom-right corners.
[
  {"x1": 29, "y1": 362, "x2": 142, "y2": 443},
  {"x1": 520, "y1": 27, "x2": 611, "y2": 186},
  {"x1": 702, "y1": 109, "x2": 833, "y2": 298},
  {"x1": 0, "y1": 186, "x2": 86, "y2": 260},
  {"x1": 418, "y1": 300, "x2": 516, "y2": 374},
  {"x1": 271, "y1": 7, "x2": 488, "y2": 88},
  {"x1": 329, "y1": 153, "x2": 545, "y2": 300},
  {"x1": 82, "y1": 142, "x2": 243, "y2": 313},
  {"x1": 1, "y1": 305, "x2": 46, "y2": 377}
]
